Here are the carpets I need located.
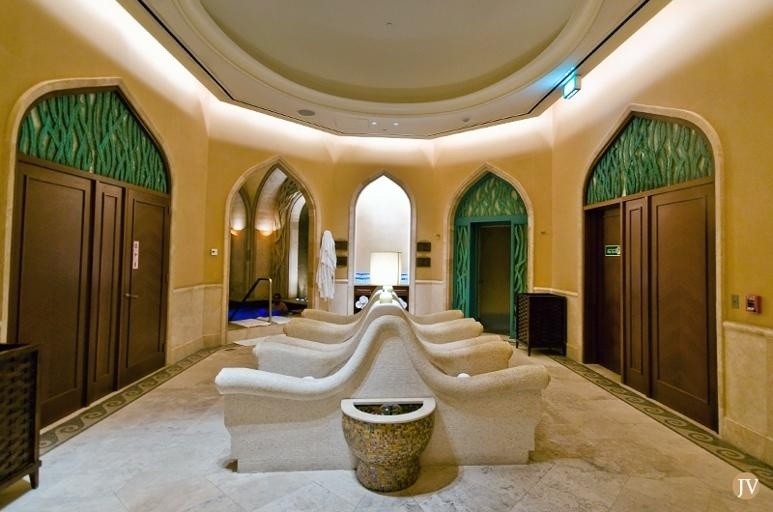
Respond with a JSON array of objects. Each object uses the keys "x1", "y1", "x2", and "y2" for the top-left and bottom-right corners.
[
  {"x1": 256, "y1": 315, "x2": 290, "y2": 325},
  {"x1": 230, "y1": 318, "x2": 271, "y2": 327}
]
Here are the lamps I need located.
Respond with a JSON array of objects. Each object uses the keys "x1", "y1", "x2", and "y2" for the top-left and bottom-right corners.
[{"x1": 369, "y1": 250, "x2": 402, "y2": 287}]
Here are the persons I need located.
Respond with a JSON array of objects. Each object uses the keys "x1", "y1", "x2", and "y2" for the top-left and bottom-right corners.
[{"x1": 271, "y1": 293, "x2": 289, "y2": 312}]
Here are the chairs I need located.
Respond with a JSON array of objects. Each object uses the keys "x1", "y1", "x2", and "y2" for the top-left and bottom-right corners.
[{"x1": 215, "y1": 281, "x2": 551, "y2": 473}]
